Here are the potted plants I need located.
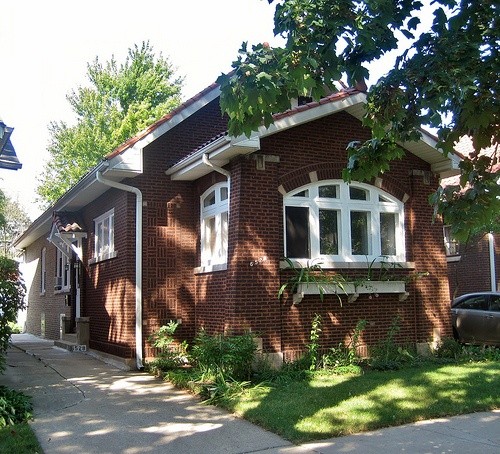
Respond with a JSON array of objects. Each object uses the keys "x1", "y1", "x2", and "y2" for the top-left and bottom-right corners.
[{"x1": 276, "y1": 254, "x2": 407, "y2": 304}]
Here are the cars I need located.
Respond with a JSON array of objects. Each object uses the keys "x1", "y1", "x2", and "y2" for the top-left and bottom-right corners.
[{"x1": 450, "y1": 291, "x2": 500, "y2": 347}]
